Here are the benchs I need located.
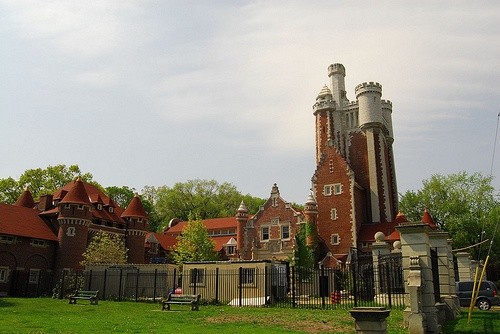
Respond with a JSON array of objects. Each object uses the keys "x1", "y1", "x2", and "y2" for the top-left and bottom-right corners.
[
  {"x1": 68, "y1": 289, "x2": 99, "y2": 305},
  {"x1": 162, "y1": 291, "x2": 201, "y2": 311}
]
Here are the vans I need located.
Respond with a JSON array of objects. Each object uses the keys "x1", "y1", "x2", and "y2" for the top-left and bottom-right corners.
[{"x1": 455, "y1": 280, "x2": 498, "y2": 311}]
